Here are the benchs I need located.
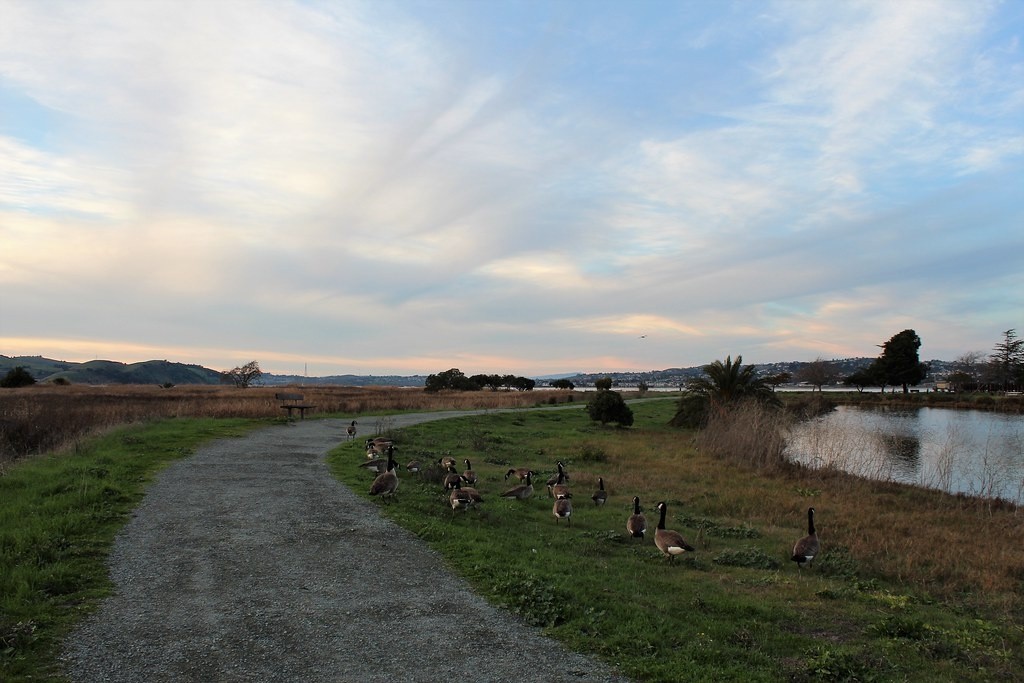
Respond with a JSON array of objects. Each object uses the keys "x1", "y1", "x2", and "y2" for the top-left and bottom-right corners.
[{"x1": 275, "y1": 392, "x2": 317, "y2": 419}]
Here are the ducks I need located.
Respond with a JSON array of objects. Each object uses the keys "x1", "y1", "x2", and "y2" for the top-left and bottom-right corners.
[
  {"x1": 627, "y1": 495, "x2": 647, "y2": 541},
  {"x1": 791, "y1": 507, "x2": 819, "y2": 572},
  {"x1": 654, "y1": 502, "x2": 696, "y2": 562},
  {"x1": 346, "y1": 420, "x2": 574, "y2": 526},
  {"x1": 590, "y1": 477, "x2": 608, "y2": 507}
]
